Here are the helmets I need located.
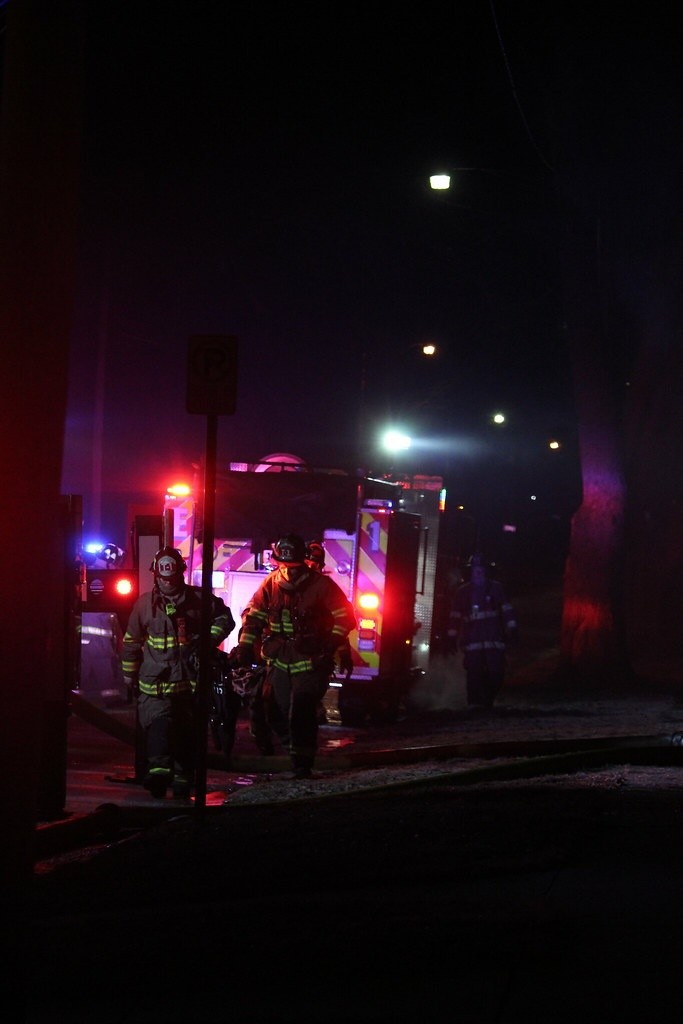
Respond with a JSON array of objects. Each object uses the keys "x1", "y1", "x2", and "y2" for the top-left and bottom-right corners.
[
  {"x1": 304, "y1": 541, "x2": 325, "y2": 565},
  {"x1": 271, "y1": 534, "x2": 306, "y2": 564},
  {"x1": 150, "y1": 546, "x2": 187, "y2": 581},
  {"x1": 466, "y1": 551, "x2": 491, "y2": 567}
]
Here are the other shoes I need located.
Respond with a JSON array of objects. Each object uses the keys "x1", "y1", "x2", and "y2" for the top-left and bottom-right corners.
[
  {"x1": 175, "y1": 778, "x2": 194, "y2": 802},
  {"x1": 283, "y1": 730, "x2": 293, "y2": 752},
  {"x1": 294, "y1": 764, "x2": 313, "y2": 779},
  {"x1": 143, "y1": 774, "x2": 168, "y2": 799}
]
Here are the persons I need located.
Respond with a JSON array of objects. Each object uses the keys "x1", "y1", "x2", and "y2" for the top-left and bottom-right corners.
[
  {"x1": 121, "y1": 547, "x2": 235, "y2": 799},
  {"x1": 447, "y1": 551, "x2": 516, "y2": 711},
  {"x1": 232, "y1": 533, "x2": 355, "y2": 778}
]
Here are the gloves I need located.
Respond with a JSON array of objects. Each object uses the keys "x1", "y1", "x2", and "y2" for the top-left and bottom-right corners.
[
  {"x1": 335, "y1": 651, "x2": 353, "y2": 680},
  {"x1": 233, "y1": 642, "x2": 255, "y2": 668},
  {"x1": 117, "y1": 671, "x2": 139, "y2": 705},
  {"x1": 316, "y1": 633, "x2": 344, "y2": 664},
  {"x1": 189, "y1": 642, "x2": 214, "y2": 672}
]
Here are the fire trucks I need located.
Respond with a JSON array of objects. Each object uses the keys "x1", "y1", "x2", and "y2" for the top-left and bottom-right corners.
[{"x1": 150, "y1": 448, "x2": 448, "y2": 733}]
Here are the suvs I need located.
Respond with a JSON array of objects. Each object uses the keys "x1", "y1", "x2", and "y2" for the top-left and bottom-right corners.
[{"x1": 64, "y1": 490, "x2": 139, "y2": 636}]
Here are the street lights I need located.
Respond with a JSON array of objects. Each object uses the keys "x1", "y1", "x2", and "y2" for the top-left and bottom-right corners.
[{"x1": 353, "y1": 339, "x2": 442, "y2": 614}]
